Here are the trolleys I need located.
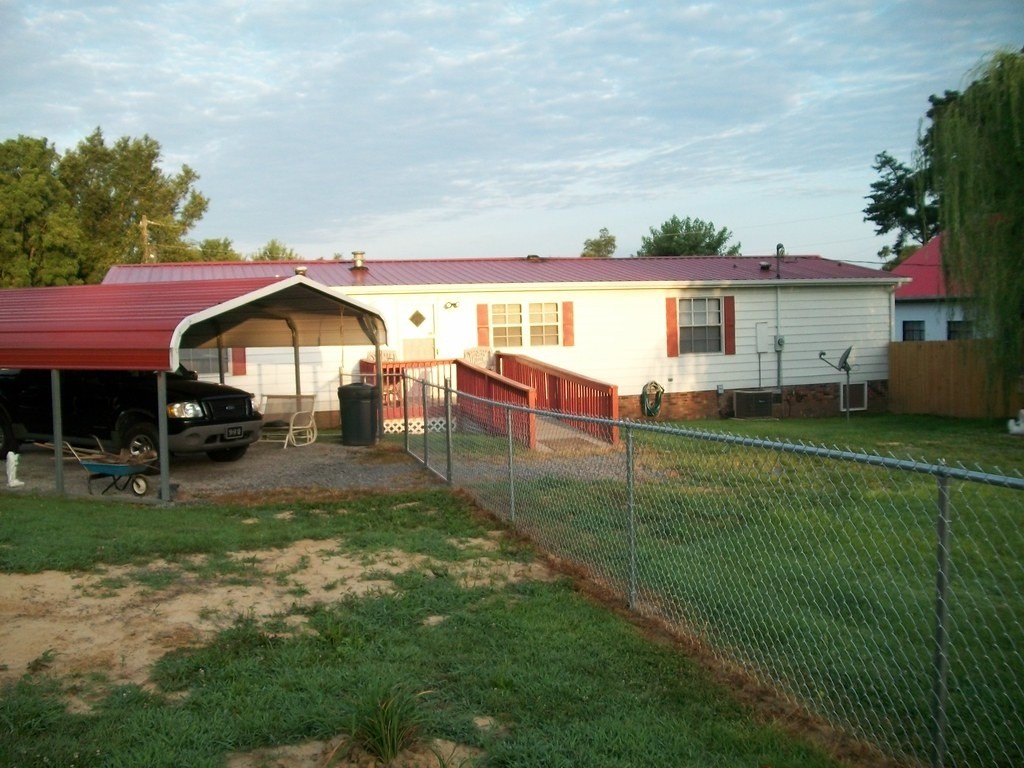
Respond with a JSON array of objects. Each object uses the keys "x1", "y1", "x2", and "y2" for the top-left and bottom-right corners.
[{"x1": 34, "y1": 439, "x2": 159, "y2": 497}]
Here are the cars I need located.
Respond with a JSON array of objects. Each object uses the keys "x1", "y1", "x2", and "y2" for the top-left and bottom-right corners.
[{"x1": 0, "y1": 360, "x2": 262, "y2": 466}]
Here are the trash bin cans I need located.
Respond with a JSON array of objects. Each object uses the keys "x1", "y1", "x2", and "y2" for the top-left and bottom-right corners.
[{"x1": 337, "y1": 382, "x2": 378, "y2": 448}]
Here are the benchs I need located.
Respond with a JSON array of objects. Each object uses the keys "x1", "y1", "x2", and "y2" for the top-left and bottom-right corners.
[{"x1": 248, "y1": 394, "x2": 318, "y2": 450}]
